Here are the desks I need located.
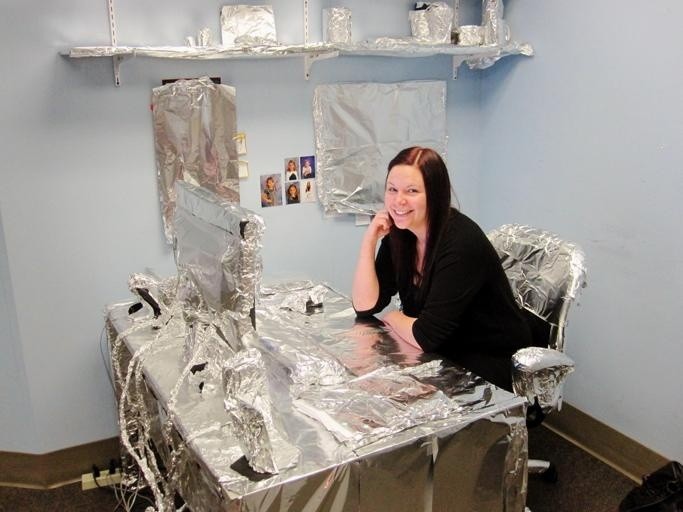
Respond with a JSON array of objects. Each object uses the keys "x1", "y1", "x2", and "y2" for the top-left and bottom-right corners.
[{"x1": 109, "y1": 282, "x2": 528, "y2": 511}]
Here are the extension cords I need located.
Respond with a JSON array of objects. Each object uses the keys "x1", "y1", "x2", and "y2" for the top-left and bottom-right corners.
[{"x1": 80, "y1": 467, "x2": 127, "y2": 491}]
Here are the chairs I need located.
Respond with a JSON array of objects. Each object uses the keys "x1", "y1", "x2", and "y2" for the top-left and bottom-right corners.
[{"x1": 488, "y1": 226, "x2": 585, "y2": 482}]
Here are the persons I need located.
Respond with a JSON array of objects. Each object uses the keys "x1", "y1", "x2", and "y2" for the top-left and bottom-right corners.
[
  {"x1": 287, "y1": 185, "x2": 298, "y2": 204},
  {"x1": 285, "y1": 160, "x2": 297, "y2": 180},
  {"x1": 262, "y1": 177, "x2": 277, "y2": 203},
  {"x1": 351, "y1": 146, "x2": 534, "y2": 393},
  {"x1": 302, "y1": 160, "x2": 312, "y2": 178}
]
